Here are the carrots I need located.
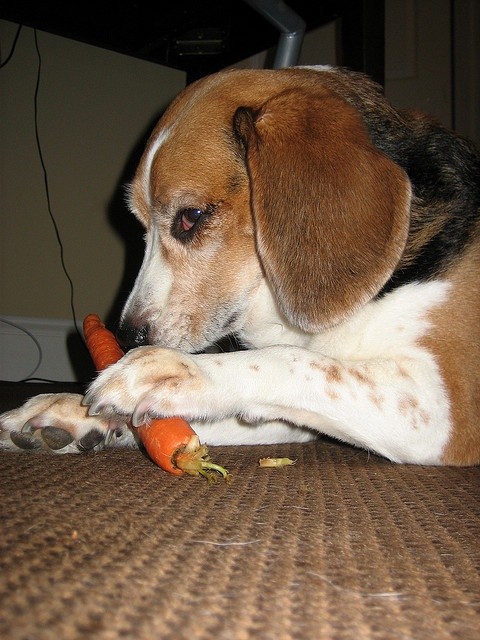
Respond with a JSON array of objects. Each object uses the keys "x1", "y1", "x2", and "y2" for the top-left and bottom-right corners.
[{"x1": 82, "y1": 313, "x2": 233, "y2": 485}]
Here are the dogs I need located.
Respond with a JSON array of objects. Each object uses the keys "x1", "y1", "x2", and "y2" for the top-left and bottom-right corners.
[{"x1": 0, "y1": 63, "x2": 480, "y2": 466}]
